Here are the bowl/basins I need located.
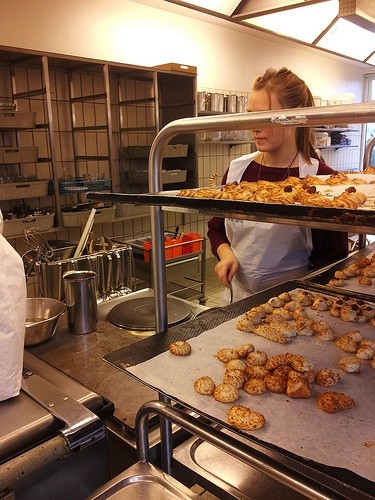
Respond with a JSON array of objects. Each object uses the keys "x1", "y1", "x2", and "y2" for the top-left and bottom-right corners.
[
  {"x1": 3, "y1": 204, "x2": 50, "y2": 220},
  {"x1": 23, "y1": 298, "x2": 65, "y2": 345},
  {"x1": 82, "y1": 173, "x2": 105, "y2": 180}
]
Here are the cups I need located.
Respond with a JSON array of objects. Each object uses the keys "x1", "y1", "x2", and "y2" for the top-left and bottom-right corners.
[{"x1": 62, "y1": 271, "x2": 97, "y2": 335}]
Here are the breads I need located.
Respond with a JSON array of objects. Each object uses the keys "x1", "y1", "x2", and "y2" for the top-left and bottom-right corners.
[
  {"x1": 176, "y1": 166, "x2": 375, "y2": 209},
  {"x1": 170, "y1": 291, "x2": 375, "y2": 431},
  {"x1": 329, "y1": 252, "x2": 375, "y2": 286}
]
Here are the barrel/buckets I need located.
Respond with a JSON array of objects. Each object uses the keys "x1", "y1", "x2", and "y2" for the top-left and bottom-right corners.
[{"x1": 315, "y1": 132, "x2": 328, "y2": 146}]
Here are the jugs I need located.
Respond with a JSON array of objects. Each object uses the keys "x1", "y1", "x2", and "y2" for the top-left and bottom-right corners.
[{"x1": 196, "y1": 91, "x2": 247, "y2": 113}]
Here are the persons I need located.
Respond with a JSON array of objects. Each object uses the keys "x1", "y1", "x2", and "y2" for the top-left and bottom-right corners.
[{"x1": 207, "y1": 68, "x2": 349, "y2": 302}]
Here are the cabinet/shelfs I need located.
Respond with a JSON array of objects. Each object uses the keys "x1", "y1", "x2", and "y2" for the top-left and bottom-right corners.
[{"x1": 0, "y1": 44, "x2": 375, "y2": 500}]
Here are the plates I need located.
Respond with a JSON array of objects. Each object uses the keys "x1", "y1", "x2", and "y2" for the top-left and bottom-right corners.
[
  {"x1": 0, "y1": 175, "x2": 40, "y2": 183},
  {"x1": 64, "y1": 186, "x2": 88, "y2": 190}
]
